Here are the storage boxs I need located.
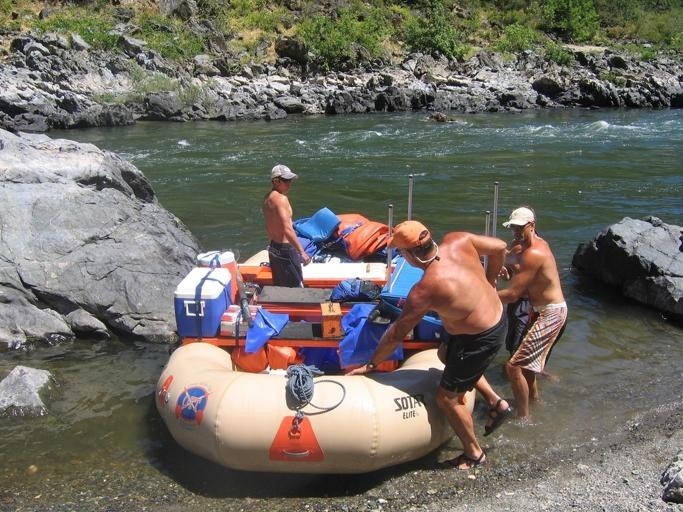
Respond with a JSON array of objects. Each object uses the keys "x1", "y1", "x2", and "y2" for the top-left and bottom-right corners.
[
  {"x1": 174, "y1": 251, "x2": 240, "y2": 338},
  {"x1": 381, "y1": 255, "x2": 450, "y2": 343}
]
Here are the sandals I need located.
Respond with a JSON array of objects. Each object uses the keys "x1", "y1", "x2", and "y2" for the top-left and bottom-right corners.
[
  {"x1": 454, "y1": 450, "x2": 487, "y2": 472},
  {"x1": 483, "y1": 398, "x2": 515, "y2": 437}
]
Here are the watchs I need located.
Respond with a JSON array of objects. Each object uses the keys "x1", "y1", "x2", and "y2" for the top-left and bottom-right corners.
[{"x1": 367, "y1": 359, "x2": 376, "y2": 371}]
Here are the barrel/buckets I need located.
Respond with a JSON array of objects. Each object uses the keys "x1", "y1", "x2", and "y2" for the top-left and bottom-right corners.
[{"x1": 198, "y1": 251, "x2": 238, "y2": 304}]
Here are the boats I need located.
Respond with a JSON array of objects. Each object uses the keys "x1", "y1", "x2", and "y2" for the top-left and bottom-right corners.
[{"x1": 154, "y1": 206, "x2": 475, "y2": 473}]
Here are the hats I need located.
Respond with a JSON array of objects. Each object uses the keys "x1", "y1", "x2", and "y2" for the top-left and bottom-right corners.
[
  {"x1": 387, "y1": 220, "x2": 430, "y2": 250},
  {"x1": 506, "y1": 208, "x2": 535, "y2": 229},
  {"x1": 271, "y1": 164, "x2": 296, "y2": 180}
]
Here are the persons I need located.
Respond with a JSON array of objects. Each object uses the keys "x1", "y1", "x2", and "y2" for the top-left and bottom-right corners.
[
  {"x1": 496, "y1": 205, "x2": 567, "y2": 421},
  {"x1": 344, "y1": 220, "x2": 514, "y2": 471},
  {"x1": 498, "y1": 232, "x2": 566, "y2": 381},
  {"x1": 261, "y1": 164, "x2": 311, "y2": 288}
]
